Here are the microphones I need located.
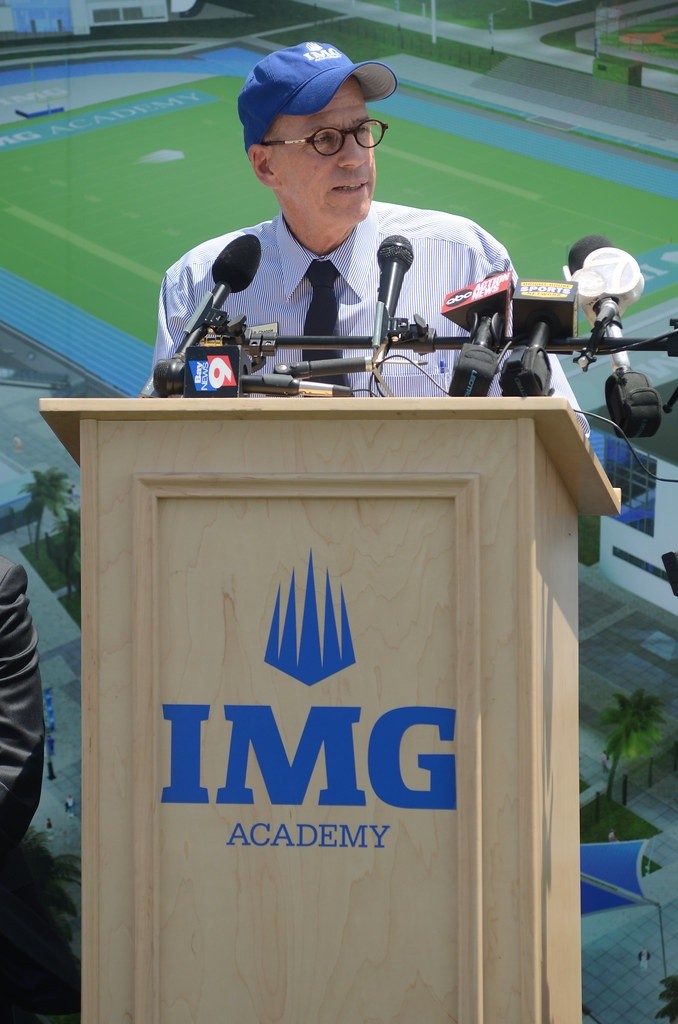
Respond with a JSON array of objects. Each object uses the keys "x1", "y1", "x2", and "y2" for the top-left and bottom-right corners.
[{"x1": 153, "y1": 234, "x2": 662, "y2": 438}]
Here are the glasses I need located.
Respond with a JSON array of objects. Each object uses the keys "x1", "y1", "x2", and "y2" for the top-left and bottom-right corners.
[{"x1": 261, "y1": 121, "x2": 388, "y2": 157}]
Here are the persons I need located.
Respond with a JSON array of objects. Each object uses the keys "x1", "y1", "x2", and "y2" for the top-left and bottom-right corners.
[
  {"x1": 0, "y1": 551, "x2": 46, "y2": 863},
  {"x1": 141, "y1": 42, "x2": 591, "y2": 439}
]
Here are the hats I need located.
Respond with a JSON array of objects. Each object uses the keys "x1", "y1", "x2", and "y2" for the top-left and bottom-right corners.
[{"x1": 238, "y1": 41, "x2": 398, "y2": 156}]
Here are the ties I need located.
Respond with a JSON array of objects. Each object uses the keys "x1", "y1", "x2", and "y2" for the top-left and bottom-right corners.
[{"x1": 300, "y1": 260, "x2": 342, "y2": 385}]
